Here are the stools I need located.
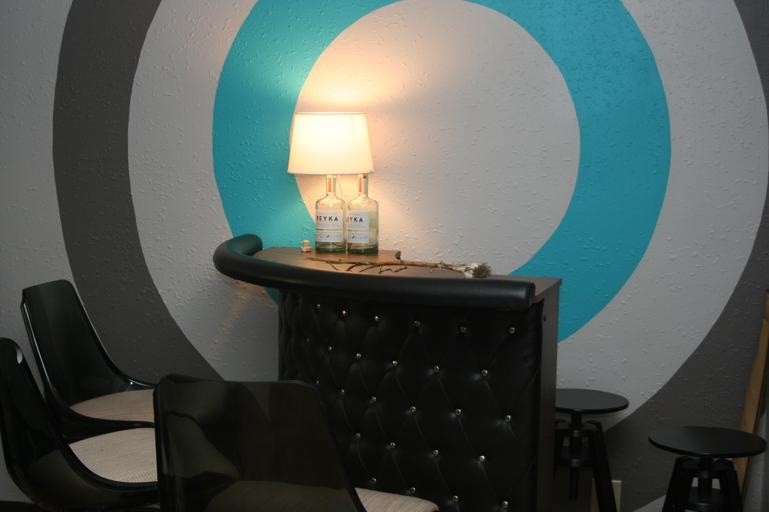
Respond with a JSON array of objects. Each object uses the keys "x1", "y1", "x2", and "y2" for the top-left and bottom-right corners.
[
  {"x1": 555, "y1": 389, "x2": 629, "y2": 512},
  {"x1": 647, "y1": 426, "x2": 767, "y2": 512}
]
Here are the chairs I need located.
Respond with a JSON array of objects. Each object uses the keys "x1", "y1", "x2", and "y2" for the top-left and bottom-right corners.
[
  {"x1": 2, "y1": 338, "x2": 158, "y2": 510},
  {"x1": 152, "y1": 373, "x2": 438, "y2": 512},
  {"x1": 21, "y1": 279, "x2": 156, "y2": 434}
]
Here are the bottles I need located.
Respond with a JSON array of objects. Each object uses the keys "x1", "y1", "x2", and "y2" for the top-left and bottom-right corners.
[
  {"x1": 346, "y1": 174, "x2": 378, "y2": 254},
  {"x1": 316, "y1": 174, "x2": 346, "y2": 253}
]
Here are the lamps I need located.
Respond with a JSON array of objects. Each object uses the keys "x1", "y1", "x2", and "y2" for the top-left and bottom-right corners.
[{"x1": 285, "y1": 112, "x2": 374, "y2": 174}]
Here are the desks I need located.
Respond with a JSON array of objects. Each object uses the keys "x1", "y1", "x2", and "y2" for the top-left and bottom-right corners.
[{"x1": 214, "y1": 234, "x2": 559, "y2": 512}]
